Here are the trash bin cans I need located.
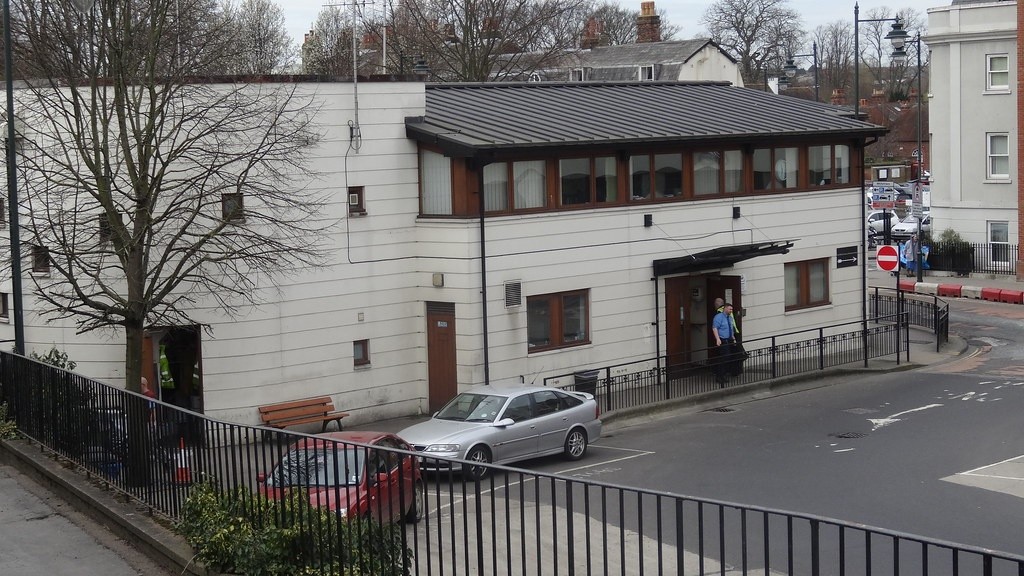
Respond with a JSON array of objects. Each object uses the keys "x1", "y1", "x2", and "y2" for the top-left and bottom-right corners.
[{"x1": 573, "y1": 371, "x2": 600, "y2": 400}]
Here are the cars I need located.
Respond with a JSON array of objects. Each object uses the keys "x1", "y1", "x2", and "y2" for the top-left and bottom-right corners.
[
  {"x1": 254, "y1": 432, "x2": 424, "y2": 537},
  {"x1": 868, "y1": 210, "x2": 899, "y2": 235},
  {"x1": 867, "y1": 169, "x2": 930, "y2": 217},
  {"x1": 890, "y1": 212, "x2": 930, "y2": 241},
  {"x1": 395, "y1": 381, "x2": 604, "y2": 480}
]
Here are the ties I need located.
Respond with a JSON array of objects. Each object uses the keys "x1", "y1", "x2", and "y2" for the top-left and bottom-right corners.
[{"x1": 727, "y1": 315, "x2": 732, "y2": 338}]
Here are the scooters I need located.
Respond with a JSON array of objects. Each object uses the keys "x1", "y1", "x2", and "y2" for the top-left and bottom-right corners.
[{"x1": 869, "y1": 224, "x2": 879, "y2": 247}]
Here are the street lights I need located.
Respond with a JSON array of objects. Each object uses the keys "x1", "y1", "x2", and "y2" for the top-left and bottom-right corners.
[
  {"x1": 3, "y1": 0, "x2": 96, "y2": 352},
  {"x1": 764, "y1": 73, "x2": 791, "y2": 92},
  {"x1": 892, "y1": 41, "x2": 923, "y2": 282},
  {"x1": 855, "y1": 16, "x2": 912, "y2": 120},
  {"x1": 785, "y1": 53, "x2": 819, "y2": 101},
  {"x1": 399, "y1": 53, "x2": 431, "y2": 80}
]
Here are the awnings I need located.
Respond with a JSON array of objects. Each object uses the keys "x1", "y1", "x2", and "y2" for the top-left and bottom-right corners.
[{"x1": 653, "y1": 239, "x2": 800, "y2": 276}]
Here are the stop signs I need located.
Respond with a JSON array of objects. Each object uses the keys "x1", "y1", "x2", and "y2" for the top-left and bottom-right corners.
[{"x1": 876, "y1": 245, "x2": 898, "y2": 271}]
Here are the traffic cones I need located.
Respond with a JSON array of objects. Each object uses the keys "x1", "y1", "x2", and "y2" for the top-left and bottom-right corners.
[{"x1": 175, "y1": 438, "x2": 196, "y2": 486}]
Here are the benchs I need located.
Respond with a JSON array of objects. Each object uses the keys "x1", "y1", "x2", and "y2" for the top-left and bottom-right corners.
[{"x1": 258, "y1": 396, "x2": 350, "y2": 437}]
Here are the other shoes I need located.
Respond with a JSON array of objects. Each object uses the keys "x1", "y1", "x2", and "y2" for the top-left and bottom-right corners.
[
  {"x1": 716, "y1": 377, "x2": 724, "y2": 384},
  {"x1": 907, "y1": 273, "x2": 916, "y2": 277}
]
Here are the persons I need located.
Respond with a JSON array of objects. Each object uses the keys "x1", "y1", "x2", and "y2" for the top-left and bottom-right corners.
[
  {"x1": 141, "y1": 377, "x2": 168, "y2": 465},
  {"x1": 712, "y1": 303, "x2": 737, "y2": 383},
  {"x1": 903, "y1": 234, "x2": 917, "y2": 277},
  {"x1": 712, "y1": 298, "x2": 739, "y2": 378}
]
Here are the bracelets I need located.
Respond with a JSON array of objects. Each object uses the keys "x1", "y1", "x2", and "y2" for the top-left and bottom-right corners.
[{"x1": 733, "y1": 339, "x2": 736, "y2": 341}]
